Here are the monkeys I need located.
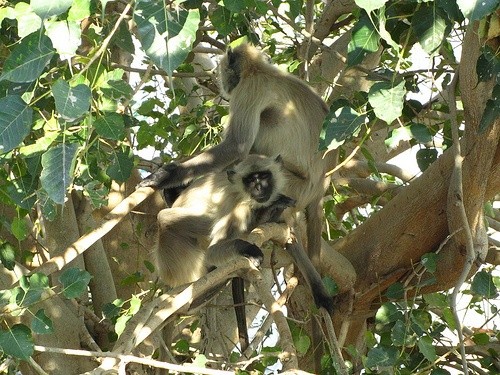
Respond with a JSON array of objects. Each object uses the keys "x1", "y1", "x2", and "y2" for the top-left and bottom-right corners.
[{"x1": 0, "y1": 39, "x2": 343, "y2": 305}]
